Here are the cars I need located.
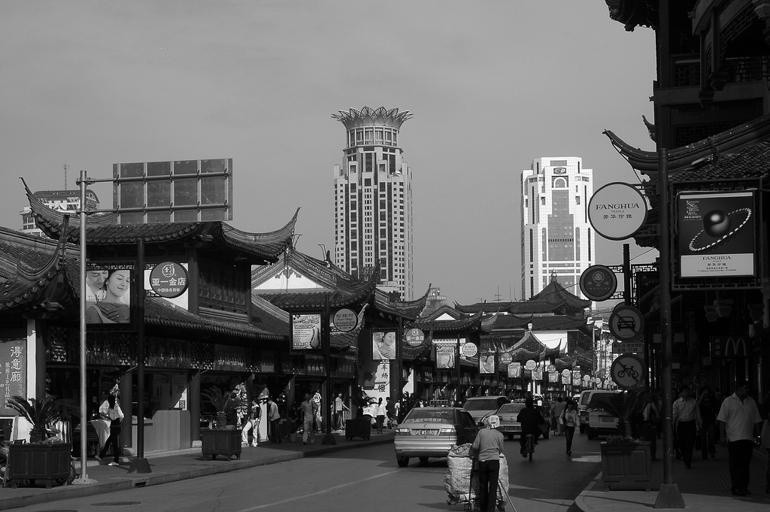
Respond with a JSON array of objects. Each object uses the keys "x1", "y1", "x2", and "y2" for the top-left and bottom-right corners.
[
  {"x1": 494, "y1": 402, "x2": 552, "y2": 440},
  {"x1": 392, "y1": 405, "x2": 485, "y2": 468}
]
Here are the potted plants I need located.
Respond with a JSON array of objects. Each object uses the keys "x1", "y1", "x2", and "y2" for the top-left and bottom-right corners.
[
  {"x1": 341, "y1": 389, "x2": 378, "y2": 440},
  {"x1": 6, "y1": 395, "x2": 71, "y2": 489},
  {"x1": 586, "y1": 386, "x2": 661, "y2": 491},
  {"x1": 200, "y1": 384, "x2": 242, "y2": 461}
]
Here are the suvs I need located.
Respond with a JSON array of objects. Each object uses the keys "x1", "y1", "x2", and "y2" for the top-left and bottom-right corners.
[
  {"x1": 462, "y1": 395, "x2": 511, "y2": 431},
  {"x1": 573, "y1": 388, "x2": 630, "y2": 439}
]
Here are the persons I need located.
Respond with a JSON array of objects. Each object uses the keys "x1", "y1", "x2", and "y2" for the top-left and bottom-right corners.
[
  {"x1": 672, "y1": 380, "x2": 762, "y2": 497},
  {"x1": 510, "y1": 391, "x2": 579, "y2": 458},
  {"x1": 85, "y1": 270, "x2": 130, "y2": 324},
  {"x1": 472, "y1": 415, "x2": 504, "y2": 512},
  {"x1": 94, "y1": 394, "x2": 124, "y2": 467},
  {"x1": 373, "y1": 331, "x2": 395, "y2": 360},
  {"x1": 223, "y1": 387, "x2": 401, "y2": 448}
]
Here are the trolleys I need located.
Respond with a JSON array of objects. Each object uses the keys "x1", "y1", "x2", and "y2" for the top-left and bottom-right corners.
[{"x1": 449, "y1": 458, "x2": 508, "y2": 510}]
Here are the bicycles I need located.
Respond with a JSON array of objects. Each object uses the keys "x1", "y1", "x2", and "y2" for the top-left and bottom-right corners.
[{"x1": 526, "y1": 433, "x2": 538, "y2": 462}]
